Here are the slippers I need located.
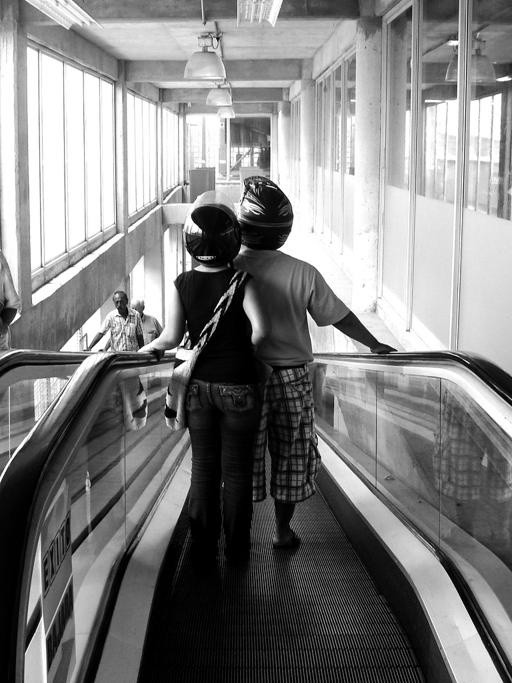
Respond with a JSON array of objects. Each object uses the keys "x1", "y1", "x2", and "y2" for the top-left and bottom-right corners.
[{"x1": 272, "y1": 531, "x2": 302, "y2": 549}]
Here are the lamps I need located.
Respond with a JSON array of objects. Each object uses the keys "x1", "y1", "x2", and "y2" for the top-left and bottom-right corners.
[
  {"x1": 444, "y1": 32, "x2": 497, "y2": 87},
  {"x1": 27, "y1": 0, "x2": 103, "y2": 31},
  {"x1": 183, "y1": 32, "x2": 237, "y2": 119},
  {"x1": 236, "y1": 0, "x2": 283, "y2": 27}
]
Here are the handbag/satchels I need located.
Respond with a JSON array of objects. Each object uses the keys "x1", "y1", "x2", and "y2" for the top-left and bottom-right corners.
[{"x1": 164, "y1": 345, "x2": 199, "y2": 431}]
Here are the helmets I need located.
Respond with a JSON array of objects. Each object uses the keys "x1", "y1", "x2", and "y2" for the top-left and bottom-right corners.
[
  {"x1": 183, "y1": 190, "x2": 242, "y2": 265},
  {"x1": 237, "y1": 175, "x2": 294, "y2": 250}
]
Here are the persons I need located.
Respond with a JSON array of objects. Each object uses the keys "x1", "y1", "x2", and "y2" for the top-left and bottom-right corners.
[
  {"x1": 1, "y1": 247, "x2": 23, "y2": 350},
  {"x1": 228, "y1": 173, "x2": 398, "y2": 548},
  {"x1": 96, "y1": 298, "x2": 164, "y2": 356},
  {"x1": 433, "y1": 387, "x2": 511, "y2": 563},
  {"x1": 86, "y1": 379, "x2": 128, "y2": 556},
  {"x1": 84, "y1": 290, "x2": 146, "y2": 354},
  {"x1": 136, "y1": 190, "x2": 272, "y2": 565}
]
